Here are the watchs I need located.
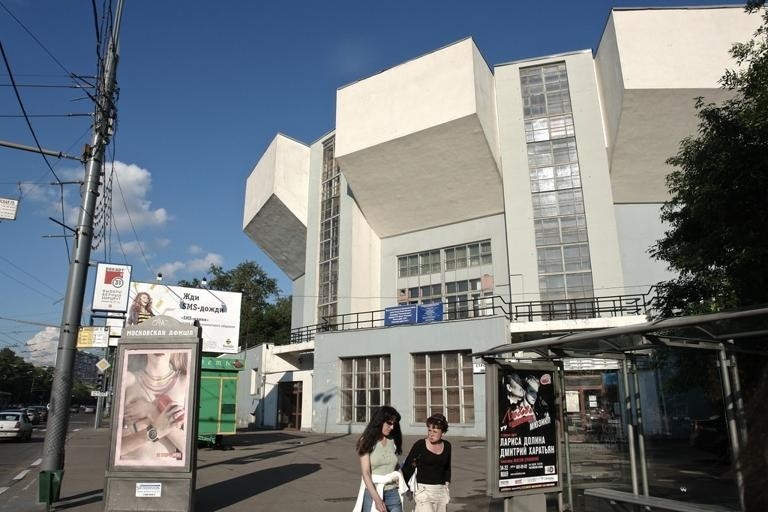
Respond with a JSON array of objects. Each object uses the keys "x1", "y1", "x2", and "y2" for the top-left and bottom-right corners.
[{"x1": 146, "y1": 424, "x2": 159, "y2": 443}]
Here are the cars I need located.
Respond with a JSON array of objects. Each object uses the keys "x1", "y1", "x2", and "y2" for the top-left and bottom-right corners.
[
  {"x1": 0, "y1": 403, "x2": 49, "y2": 441},
  {"x1": 70, "y1": 405, "x2": 96, "y2": 413}
]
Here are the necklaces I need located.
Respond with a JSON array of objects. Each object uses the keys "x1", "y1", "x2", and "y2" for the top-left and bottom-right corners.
[{"x1": 136, "y1": 364, "x2": 176, "y2": 403}]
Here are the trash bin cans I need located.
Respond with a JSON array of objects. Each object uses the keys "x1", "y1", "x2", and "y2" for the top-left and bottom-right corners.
[
  {"x1": 248, "y1": 423, "x2": 255, "y2": 431},
  {"x1": 39, "y1": 470, "x2": 65, "y2": 512}
]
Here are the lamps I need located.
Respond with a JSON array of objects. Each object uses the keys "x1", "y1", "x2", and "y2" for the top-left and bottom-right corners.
[
  {"x1": 156, "y1": 272, "x2": 163, "y2": 284},
  {"x1": 202, "y1": 276, "x2": 207, "y2": 288}
]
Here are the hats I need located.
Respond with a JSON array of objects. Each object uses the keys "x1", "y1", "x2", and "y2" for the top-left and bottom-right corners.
[{"x1": 426, "y1": 413, "x2": 449, "y2": 428}]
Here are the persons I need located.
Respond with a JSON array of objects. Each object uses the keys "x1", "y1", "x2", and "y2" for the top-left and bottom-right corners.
[
  {"x1": 498, "y1": 371, "x2": 526, "y2": 425},
  {"x1": 506, "y1": 373, "x2": 553, "y2": 457},
  {"x1": 119, "y1": 352, "x2": 191, "y2": 465},
  {"x1": 352, "y1": 404, "x2": 405, "y2": 512},
  {"x1": 125, "y1": 291, "x2": 154, "y2": 327},
  {"x1": 403, "y1": 414, "x2": 452, "y2": 512}
]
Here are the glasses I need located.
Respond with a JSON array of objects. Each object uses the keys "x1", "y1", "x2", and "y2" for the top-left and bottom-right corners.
[{"x1": 385, "y1": 420, "x2": 397, "y2": 426}]
